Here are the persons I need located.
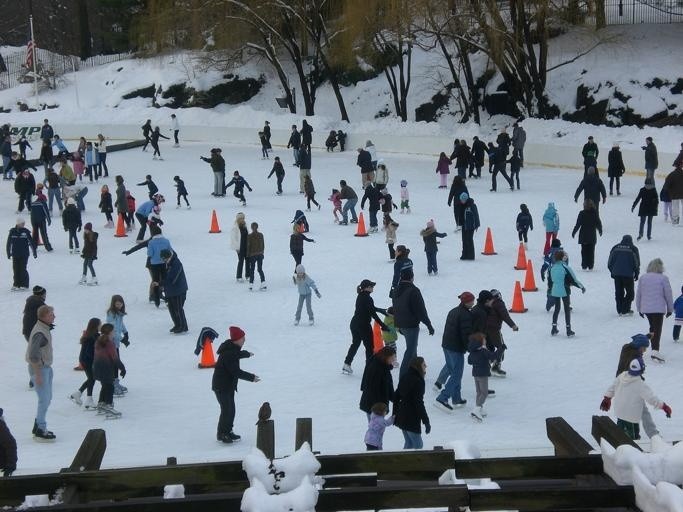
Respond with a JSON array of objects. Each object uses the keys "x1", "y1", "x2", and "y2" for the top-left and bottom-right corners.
[{"x1": 0, "y1": 109, "x2": 682, "y2": 477}]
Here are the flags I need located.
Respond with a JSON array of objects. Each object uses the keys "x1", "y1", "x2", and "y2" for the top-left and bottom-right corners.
[{"x1": 25, "y1": 16, "x2": 38, "y2": 71}]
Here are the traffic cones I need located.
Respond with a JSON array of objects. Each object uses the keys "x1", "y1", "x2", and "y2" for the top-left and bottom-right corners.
[
  {"x1": 509, "y1": 243, "x2": 538, "y2": 313},
  {"x1": 114, "y1": 213, "x2": 128, "y2": 237},
  {"x1": 209, "y1": 210, "x2": 221, "y2": 233},
  {"x1": 354, "y1": 212, "x2": 369, "y2": 236},
  {"x1": 373, "y1": 320, "x2": 384, "y2": 353},
  {"x1": 481, "y1": 227, "x2": 497, "y2": 255},
  {"x1": 198, "y1": 337, "x2": 217, "y2": 367}
]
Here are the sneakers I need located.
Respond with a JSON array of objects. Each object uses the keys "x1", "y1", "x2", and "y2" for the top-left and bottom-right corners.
[
  {"x1": 492, "y1": 365, "x2": 506, "y2": 374},
  {"x1": 32, "y1": 426, "x2": 56, "y2": 439},
  {"x1": 652, "y1": 352, "x2": 665, "y2": 360},
  {"x1": 435, "y1": 382, "x2": 495, "y2": 420},
  {"x1": 343, "y1": 365, "x2": 353, "y2": 373},
  {"x1": 79, "y1": 279, "x2": 98, "y2": 285},
  {"x1": 295, "y1": 320, "x2": 314, "y2": 325},
  {"x1": 170, "y1": 327, "x2": 188, "y2": 333},
  {"x1": 71, "y1": 384, "x2": 128, "y2": 414},
  {"x1": 551, "y1": 328, "x2": 575, "y2": 336},
  {"x1": 11, "y1": 286, "x2": 27, "y2": 291},
  {"x1": 219, "y1": 431, "x2": 240, "y2": 443},
  {"x1": 334, "y1": 217, "x2": 358, "y2": 223},
  {"x1": 237, "y1": 277, "x2": 266, "y2": 288}
]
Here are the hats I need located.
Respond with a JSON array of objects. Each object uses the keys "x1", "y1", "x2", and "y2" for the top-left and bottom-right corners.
[
  {"x1": 160, "y1": 250, "x2": 172, "y2": 259},
  {"x1": 460, "y1": 289, "x2": 500, "y2": 303},
  {"x1": 229, "y1": 326, "x2": 245, "y2": 340},
  {"x1": 68, "y1": 197, "x2": 76, "y2": 205},
  {"x1": 85, "y1": 223, "x2": 92, "y2": 230},
  {"x1": 551, "y1": 239, "x2": 561, "y2": 246},
  {"x1": 460, "y1": 192, "x2": 468, "y2": 201},
  {"x1": 357, "y1": 140, "x2": 386, "y2": 166},
  {"x1": 360, "y1": 270, "x2": 413, "y2": 288},
  {"x1": 296, "y1": 264, "x2": 305, "y2": 273},
  {"x1": 15, "y1": 217, "x2": 25, "y2": 225},
  {"x1": 426, "y1": 219, "x2": 434, "y2": 227},
  {"x1": 629, "y1": 334, "x2": 650, "y2": 376},
  {"x1": 33, "y1": 286, "x2": 46, "y2": 295}
]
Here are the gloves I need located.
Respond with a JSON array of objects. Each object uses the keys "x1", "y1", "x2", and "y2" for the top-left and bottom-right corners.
[
  {"x1": 121, "y1": 333, "x2": 131, "y2": 347},
  {"x1": 600, "y1": 397, "x2": 611, "y2": 411},
  {"x1": 120, "y1": 369, "x2": 126, "y2": 378},
  {"x1": 663, "y1": 403, "x2": 671, "y2": 417},
  {"x1": 382, "y1": 309, "x2": 389, "y2": 316},
  {"x1": 425, "y1": 424, "x2": 431, "y2": 434},
  {"x1": 428, "y1": 325, "x2": 434, "y2": 335},
  {"x1": 634, "y1": 274, "x2": 638, "y2": 281}
]
